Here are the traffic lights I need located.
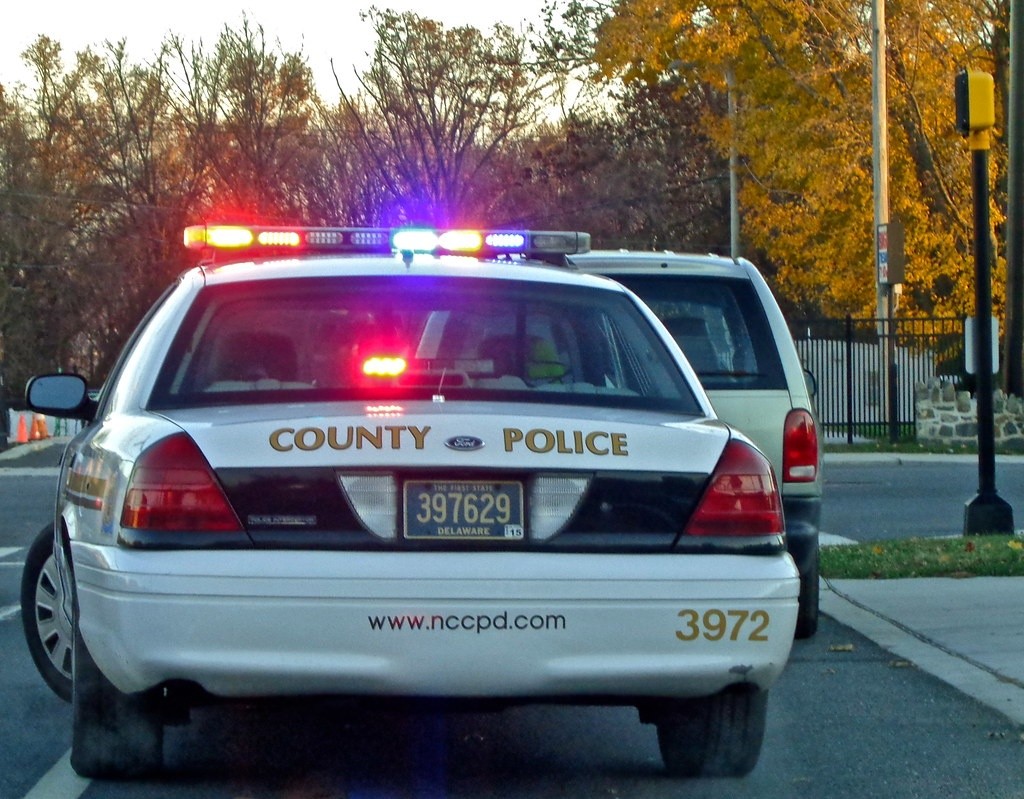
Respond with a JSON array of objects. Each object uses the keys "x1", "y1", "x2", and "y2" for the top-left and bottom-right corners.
[{"x1": 953, "y1": 64, "x2": 996, "y2": 138}]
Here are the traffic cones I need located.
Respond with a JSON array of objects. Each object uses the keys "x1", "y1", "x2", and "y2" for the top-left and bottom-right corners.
[
  {"x1": 35, "y1": 412, "x2": 50, "y2": 439},
  {"x1": 15, "y1": 415, "x2": 30, "y2": 445},
  {"x1": 26, "y1": 412, "x2": 42, "y2": 440}
]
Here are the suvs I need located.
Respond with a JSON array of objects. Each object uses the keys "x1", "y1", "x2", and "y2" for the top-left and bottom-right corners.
[{"x1": 551, "y1": 246, "x2": 830, "y2": 641}]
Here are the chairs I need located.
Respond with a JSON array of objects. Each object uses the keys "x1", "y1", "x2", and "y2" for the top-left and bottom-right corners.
[
  {"x1": 217, "y1": 334, "x2": 302, "y2": 385},
  {"x1": 663, "y1": 316, "x2": 719, "y2": 369},
  {"x1": 480, "y1": 337, "x2": 566, "y2": 384}
]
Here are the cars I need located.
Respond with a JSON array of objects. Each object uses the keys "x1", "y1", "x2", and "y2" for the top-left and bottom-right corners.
[{"x1": 24, "y1": 221, "x2": 803, "y2": 783}]
